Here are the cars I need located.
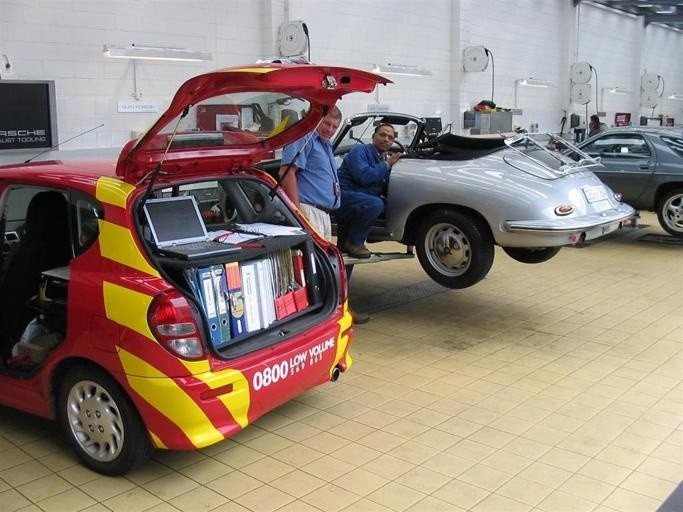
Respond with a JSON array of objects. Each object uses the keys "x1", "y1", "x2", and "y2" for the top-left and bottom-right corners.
[{"x1": 0, "y1": 61, "x2": 393, "y2": 477}]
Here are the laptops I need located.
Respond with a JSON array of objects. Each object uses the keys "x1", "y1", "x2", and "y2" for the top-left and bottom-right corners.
[{"x1": 141, "y1": 196, "x2": 242, "y2": 261}]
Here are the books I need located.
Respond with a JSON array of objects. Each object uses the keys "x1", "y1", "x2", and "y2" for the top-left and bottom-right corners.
[{"x1": 241, "y1": 248, "x2": 307, "y2": 332}]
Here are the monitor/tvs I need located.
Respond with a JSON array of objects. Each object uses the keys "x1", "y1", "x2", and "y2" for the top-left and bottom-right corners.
[{"x1": 0, "y1": 80, "x2": 59, "y2": 154}]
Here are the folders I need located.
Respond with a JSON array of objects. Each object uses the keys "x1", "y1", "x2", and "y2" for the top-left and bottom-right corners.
[{"x1": 198, "y1": 265, "x2": 231, "y2": 347}]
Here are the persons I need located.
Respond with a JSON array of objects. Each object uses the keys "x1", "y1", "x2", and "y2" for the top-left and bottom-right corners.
[
  {"x1": 337, "y1": 124, "x2": 400, "y2": 323},
  {"x1": 278, "y1": 104, "x2": 341, "y2": 241},
  {"x1": 589, "y1": 114, "x2": 600, "y2": 136}
]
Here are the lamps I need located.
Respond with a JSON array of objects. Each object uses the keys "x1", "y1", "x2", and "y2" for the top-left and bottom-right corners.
[
  {"x1": 375, "y1": 63, "x2": 432, "y2": 76},
  {"x1": 104, "y1": 43, "x2": 210, "y2": 62},
  {"x1": 610, "y1": 86, "x2": 629, "y2": 95},
  {"x1": 519, "y1": 76, "x2": 556, "y2": 89}
]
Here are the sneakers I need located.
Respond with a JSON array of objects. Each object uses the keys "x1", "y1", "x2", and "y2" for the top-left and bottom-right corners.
[
  {"x1": 341, "y1": 238, "x2": 372, "y2": 260},
  {"x1": 351, "y1": 309, "x2": 369, "y2": 324}
]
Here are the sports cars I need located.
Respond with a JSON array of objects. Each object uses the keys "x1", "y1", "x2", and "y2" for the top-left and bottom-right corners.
[
  {"x1": 559, "y1": 125, "x2": 682, "y2": 240},
  {"x1": 253, "y1": 110, "x2": 636, "y2": 289}
]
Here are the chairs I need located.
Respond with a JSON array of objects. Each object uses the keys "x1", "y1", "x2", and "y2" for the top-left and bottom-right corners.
[{"x1": 2, "y1": 191, "x2": 69, "y2": 346}]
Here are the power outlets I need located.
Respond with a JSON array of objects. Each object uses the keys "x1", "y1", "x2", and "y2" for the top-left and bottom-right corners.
[{"x1": 1, "y1": 59, "x2": 14, "y2": 74}]
